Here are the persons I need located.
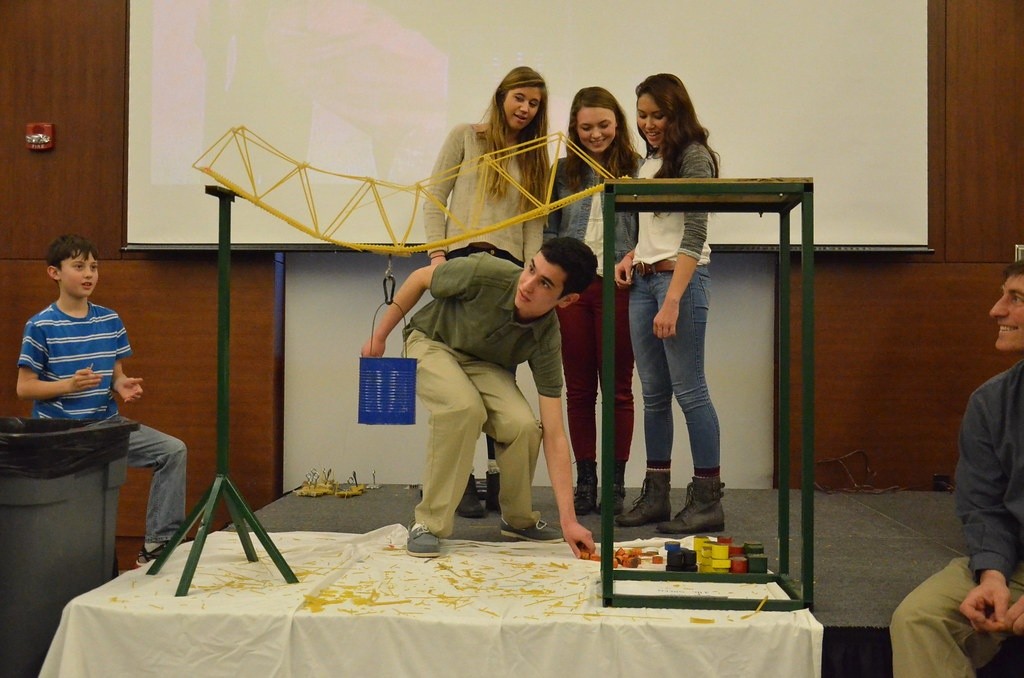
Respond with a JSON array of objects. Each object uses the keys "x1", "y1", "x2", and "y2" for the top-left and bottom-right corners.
[
  {"x1": 360, "y1": 236, "x2": 597, "y2": 558},
  {"x1": 613, "y1": 73, "x2": 725, "y2": 534},
  {"x1": 890, "y1": 260, "x2": 1024, "y2": 678},
  {"x1": 423, "y1": 66, "x2": 551, "y2": 519},
  {"x1": 17, "y1": 235, "x2": 191, "y2": 565},
  {"x1": 545, "y1": 86, "x2": 645, "y2": 516}
]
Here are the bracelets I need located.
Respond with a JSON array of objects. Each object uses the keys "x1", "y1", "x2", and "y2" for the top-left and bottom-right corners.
[
  {"x1": 430, "y1": 254, "x2": 446, "y2": 260},
  {"x1": 626, "y1": 253, "x2": 634, "y2": 261}
]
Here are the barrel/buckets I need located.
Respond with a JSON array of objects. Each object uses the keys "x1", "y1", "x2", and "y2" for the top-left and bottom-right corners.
[{"x1": 358, "y1": 302, "x2": 418, "y2": 427}]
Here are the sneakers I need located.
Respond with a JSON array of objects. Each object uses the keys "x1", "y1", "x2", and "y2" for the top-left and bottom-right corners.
[
  {"x1": 406, "y1": 519, "x2": 441, "y2": 556},
  {"x1": 499, "y1": 515, "x2": 565, "y2": 543}
]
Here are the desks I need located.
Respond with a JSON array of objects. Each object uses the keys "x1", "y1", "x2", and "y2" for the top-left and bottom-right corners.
[{"x1": 600, "y1": 174, "x2": 815, "y2": 608}]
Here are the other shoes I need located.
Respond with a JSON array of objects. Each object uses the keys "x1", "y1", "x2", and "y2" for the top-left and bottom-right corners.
[{"x1": 137, "y1": 541, "x2": 167, "y2": 566}]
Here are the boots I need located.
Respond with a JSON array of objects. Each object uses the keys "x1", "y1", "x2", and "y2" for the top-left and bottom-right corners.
[
  {"x1": 573, "y1": 461, "x2": 598, "y2": 515},
  {"x1": 655, "y1": 466, "x2": 725, "y2": 534},
  {"x1": 616, "y1": 460, "x2": 672, "y2": 526},
  {"x1": 598, "y1": 462, "x2": 626, "y2": 515},
  {"x1": 457, "y1": 466, "x2": 487, "y2": 518},
  {"x1": 486, "y1": 471, "x2": 502, "y2": 512}
]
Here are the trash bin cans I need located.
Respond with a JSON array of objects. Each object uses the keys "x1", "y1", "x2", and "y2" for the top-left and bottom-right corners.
[{"x1": 0, "y1": 419, "x2": 139, "y2": 678}]
